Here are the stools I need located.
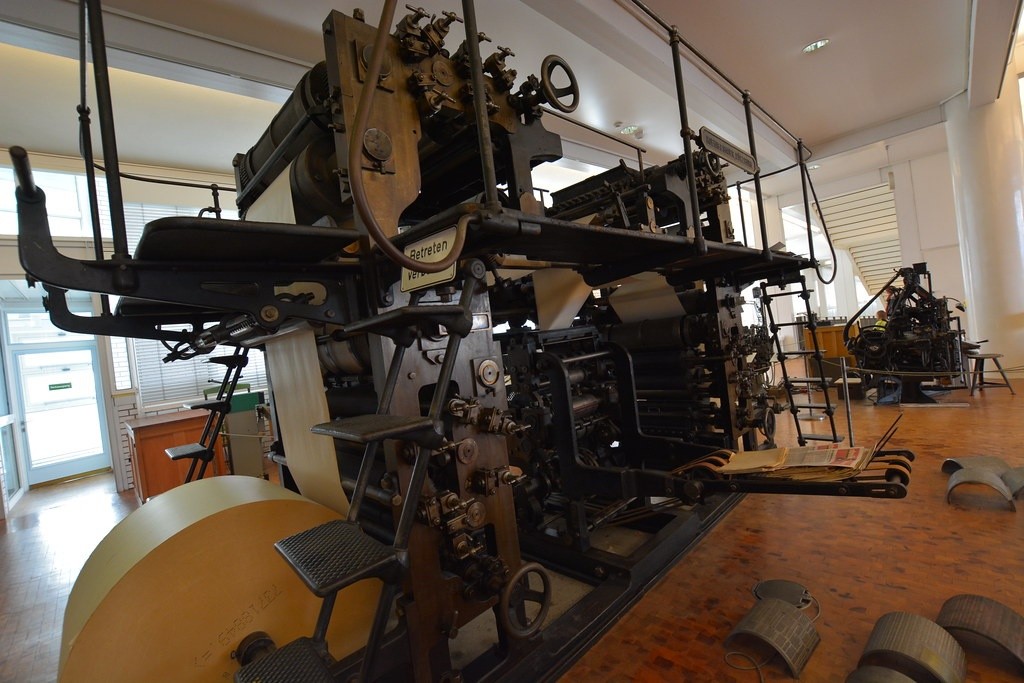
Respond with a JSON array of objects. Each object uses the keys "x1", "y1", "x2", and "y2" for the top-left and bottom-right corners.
[{"x1": 967, "y1": 354, "x2": 1016, "y2": 396}]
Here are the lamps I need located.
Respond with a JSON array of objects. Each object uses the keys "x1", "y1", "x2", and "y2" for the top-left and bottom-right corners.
[{"x1": 885, "y1": 145, "x2": 894, "y2": 190}]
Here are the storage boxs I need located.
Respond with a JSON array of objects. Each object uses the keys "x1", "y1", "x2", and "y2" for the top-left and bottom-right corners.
[{"x1": 834, "y1": 378, "x2": 866, "y2": 400}]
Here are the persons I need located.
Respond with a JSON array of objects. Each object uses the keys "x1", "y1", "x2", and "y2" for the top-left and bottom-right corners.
[{"x1": 872, "y1": 310, "x2": 887, "y2": 331}]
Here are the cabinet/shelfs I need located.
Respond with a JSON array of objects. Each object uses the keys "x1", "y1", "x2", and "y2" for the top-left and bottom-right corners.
[
  {"x1": 125, "y1": 408, "x2": 227, "y2": 507},
  {"x1": 804, "y1": 321, "x2": 861, "y2": 385}
]
